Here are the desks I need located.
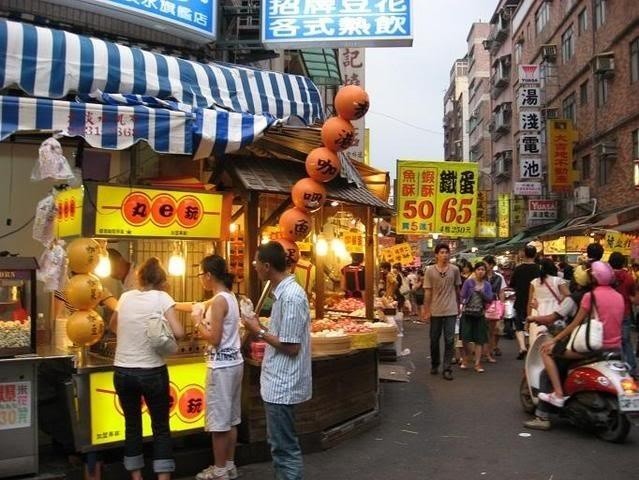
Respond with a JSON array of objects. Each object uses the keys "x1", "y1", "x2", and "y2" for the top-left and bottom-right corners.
[{"x1": 244, "y1": 343, "x2": 396, "y2": 466}]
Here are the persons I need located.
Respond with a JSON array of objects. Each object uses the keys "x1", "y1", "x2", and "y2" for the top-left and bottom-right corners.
[
  {"x1": 241, "y1": 240, "x2": 314, "y2": 480},
  {"x1": 289, "y1": 242, "x2": 639, "y2": 432},
  {"x1": 107, "y1": 257, "x2": 186, "y2": 480},
  {"x1": 95, "y1": 288, "x2": 118, "y2": 311},
  {"x1": 173, "y1": 254, "x2": 244, "y2": 480},
  {"x1": 106, "y1": 248, "x2": 140, "y2": 291}
]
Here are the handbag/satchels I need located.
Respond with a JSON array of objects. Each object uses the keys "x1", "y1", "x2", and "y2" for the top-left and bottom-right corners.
[
  {"x1": 145, "y1": 314, "x2": 179, "y2": 358},
  {"x1": 566, "y1": 293, "x2": 605, "y2": 355},
  {"x1": 482, "y1": 293, "x2": 505, "y2": 322}
]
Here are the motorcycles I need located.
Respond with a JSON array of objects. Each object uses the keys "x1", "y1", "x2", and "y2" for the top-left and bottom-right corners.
[
  {"x1": 519, "y1": 311, "x2": 639, "y2": 445},
  {"x1": 501, "y1": 290, "x2": 520, "y2": 339}
]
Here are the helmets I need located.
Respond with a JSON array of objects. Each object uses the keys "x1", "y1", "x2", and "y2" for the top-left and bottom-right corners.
[{"x1": 589, "y1": 261, "x2": 615, "y2": 285}]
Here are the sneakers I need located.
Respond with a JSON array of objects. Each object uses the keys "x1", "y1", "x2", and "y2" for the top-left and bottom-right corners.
[
  {"x1": 195, "y1": 466, "x2": 239, "y2": 479},
  {"x1": 442, "y1": 366, "x2": 453, "y2": 380},
  {"x1": 516, "y1": 350, "x2": 528, "y2": 361},
  {"x1": 522, "y1": 417, "x2": 552, "y2": 431},
  {"x1": 450, "y1": 347, "x2": 503, "y2": 373},
  {"x1": 537, "y1": 391, "x2": 565, "y2": 407},
  {"x1": 430, "y1": 364, "x2": 440, "y2": 374}
]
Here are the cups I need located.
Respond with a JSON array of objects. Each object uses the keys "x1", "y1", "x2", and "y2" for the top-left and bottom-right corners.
[{"x1": 55, "y1": 318, "x2": 67, "y2": 349}]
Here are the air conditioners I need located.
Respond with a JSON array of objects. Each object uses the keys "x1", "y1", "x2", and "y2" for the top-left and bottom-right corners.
[
  {"x1": 574, "y1": 186, "x2": 590, "y2": 205},
  {"x1": 543, "y1": 47, "x2": 557, "y2": 55},
  {"x1": 594, "y1": 57, "x2": 614, "y2": 71}
]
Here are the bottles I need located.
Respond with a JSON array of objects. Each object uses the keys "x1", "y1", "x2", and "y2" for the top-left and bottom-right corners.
[{"x1": 35, "y1": 312, "x2": 48, "y2": 356}]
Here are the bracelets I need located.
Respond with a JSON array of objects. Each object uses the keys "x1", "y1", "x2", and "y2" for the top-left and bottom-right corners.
[
  {"x1": 256, "y1": 329, "x2": 265, "y2": 340},
  {"x1": 194, "y1": 322, "x2": 202, "y2": 334}
]
